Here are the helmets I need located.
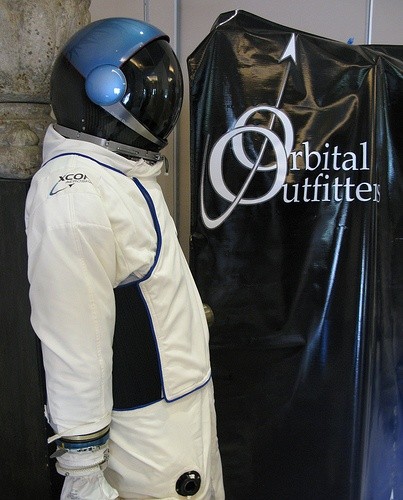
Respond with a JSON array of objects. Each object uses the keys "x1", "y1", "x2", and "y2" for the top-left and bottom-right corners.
[{"x1": 50, "y1": 18, "x2": 187, "y2": 175}]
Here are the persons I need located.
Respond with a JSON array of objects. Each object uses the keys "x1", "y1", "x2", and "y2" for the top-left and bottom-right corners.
[{"x1": 25, "y1": 18, "x2": 225, "y2": 500}]
